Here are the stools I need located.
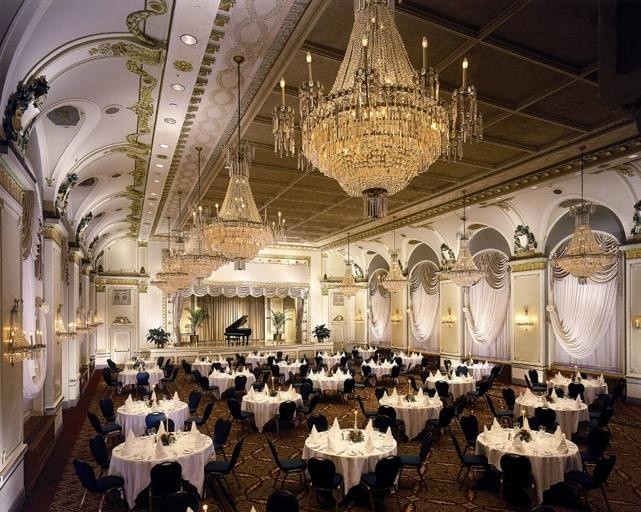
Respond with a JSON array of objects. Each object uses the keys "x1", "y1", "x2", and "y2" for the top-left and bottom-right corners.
[{"x1": 225, "y1": 337, "x2": 240, "y2": 344}]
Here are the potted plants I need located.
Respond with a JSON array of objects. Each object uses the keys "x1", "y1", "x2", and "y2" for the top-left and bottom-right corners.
[
  {"x1": 312, "y1": 322, "x2": 332, "y2": 344},
  {"x1": 183, "y1": 306, "x2": 210, "y2": 343},
  {"x1": 144, "y1": 326, "x2": 173, "y2": 349},
  {"x1": 265, "y1": 307, "x2": 294, "y2": 341}
]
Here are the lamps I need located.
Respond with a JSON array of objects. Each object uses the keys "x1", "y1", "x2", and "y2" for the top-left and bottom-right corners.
[
  {"x1": 447, "y1": 189, "x2": 481, "y2": 289},
  {"x1": 0, "y1": 296, "x2": 106, "y2": 367},
  {"x1": 267, "y1": 0, "x2": 490, "y2": 222},
  {"x1": 441, "y1": 307, "x2": 456, "y2": 328},
  {"x1": 515, "y1": 304, "x2": 535, "y2": 331},
  {"x1": 380, "y1": 215, "x2": 412, "y2": 294},
  {"x1": 332, "y1": 231, "x2": 367, "y2": 299},
  {"x1": 203, "y1": 53, "x2": 289, "y2": 273},
  {"x1": 553, "y1": 145, "x2": 616, "y2": 285},
  {"x1": 390, "y1": 309, "x2": 403, "y2": 327},
  {"x1": 151, "y1": 146, "x2": 233, "y2": 302}
]
